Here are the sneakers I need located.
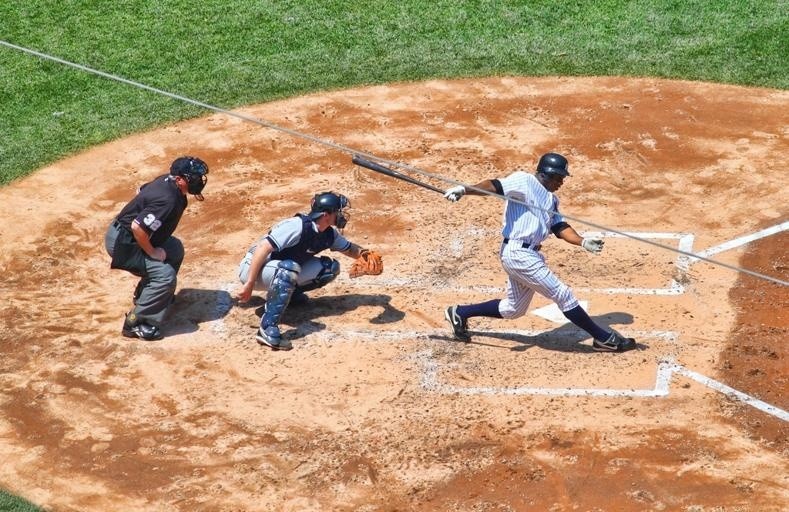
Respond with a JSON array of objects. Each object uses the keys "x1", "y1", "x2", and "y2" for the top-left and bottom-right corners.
[
  {"x1": 267, "y1": 292, "x2": 307, "y2": 305},
  {"x1": 256, "y1": 330, "x2": 292, "y2": 349},
  {"x1": 592, "y1": 331, "x2": 634, "y2": 352},
  {"x1": 123, "y1": 313, "x2": 162, "y2": 339},
  {"x1": 133, "y1": 282, "x2": 175, "y2": 305},
  {"x1": 445, "y1": 305, "x2": 470, "y2": 339}
]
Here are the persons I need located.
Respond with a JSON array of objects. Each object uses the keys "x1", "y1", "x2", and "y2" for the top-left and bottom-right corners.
[
  {"x1": 236, "y1": 190, "x2": 384, "y2": 350},
  {"x1": 444, "y1": 153, "x2": 635, "y2": 353},
  {"x1": 105, "y1": 156, "x2": 209, "y2": 341}
]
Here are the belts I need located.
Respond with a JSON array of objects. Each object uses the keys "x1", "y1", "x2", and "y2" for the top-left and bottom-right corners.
[{"x1": 504, "y1": 238, "x2": 541, "y2": 250}]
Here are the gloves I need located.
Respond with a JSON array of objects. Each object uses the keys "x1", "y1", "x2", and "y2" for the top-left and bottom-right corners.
[
  {"x1": 443, "y1": 185, "x2": 465, "y2": 202},
  {"x1": 582, "y1": 238, "x2": 604, "y2": 252}
]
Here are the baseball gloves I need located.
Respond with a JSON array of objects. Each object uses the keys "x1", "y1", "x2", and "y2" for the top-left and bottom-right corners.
[{"x1": 349, "y1": 247, "x2": 383, "y2": 279}]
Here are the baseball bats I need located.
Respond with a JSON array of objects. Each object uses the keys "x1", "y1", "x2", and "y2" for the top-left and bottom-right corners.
[{"x1": 351, "y1": 154, "x2": 458, "y2": 201}]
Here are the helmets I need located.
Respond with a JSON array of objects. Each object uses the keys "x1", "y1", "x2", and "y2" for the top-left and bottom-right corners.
[
  {"x1": 308, "y1": 190, "x2": 340, "y2": 219},
  {"x1": 537, "y1": 153, "x2": 569, "y2": 182}
]
